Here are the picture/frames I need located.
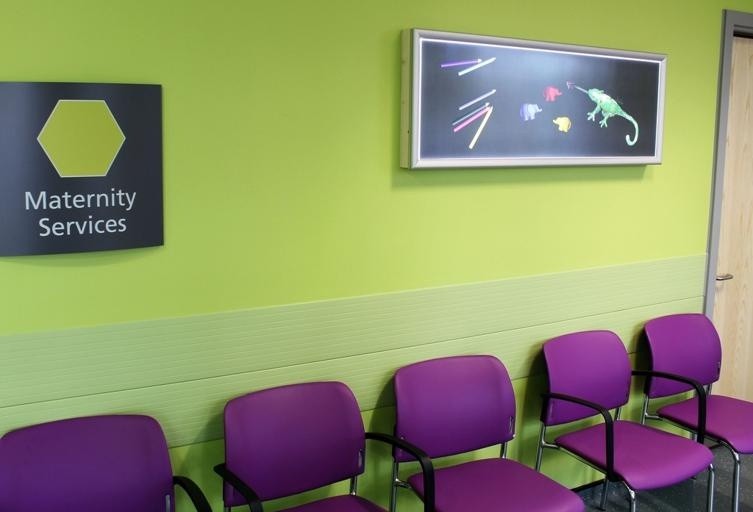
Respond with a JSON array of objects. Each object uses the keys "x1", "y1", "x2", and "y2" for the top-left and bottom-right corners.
[{"x1": 404, "y1": 27, "x2": 670, "y2": 170}]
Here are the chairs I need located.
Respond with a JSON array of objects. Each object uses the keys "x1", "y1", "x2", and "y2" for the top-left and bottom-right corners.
[
  {"x1": 1, "y1": 409, "x2": 213, "y2": 511},
  {"x1": 527, "y1": 328, "x2": 718, "y2": 511},
  {"x1": 211, "y1": 379, "x2": 441, "y2": 510},
  {"x1": 383, "y1": 352, "x2": 594, "y2": 510},
  {"x1": 637, "y1": 310, "x2": 751, "y2": 511}
]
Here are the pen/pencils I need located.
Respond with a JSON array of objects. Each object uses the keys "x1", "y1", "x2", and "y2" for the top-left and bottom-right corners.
[{"x1": 440, "y1": 56, "x2": 498, "y2": 149}]
